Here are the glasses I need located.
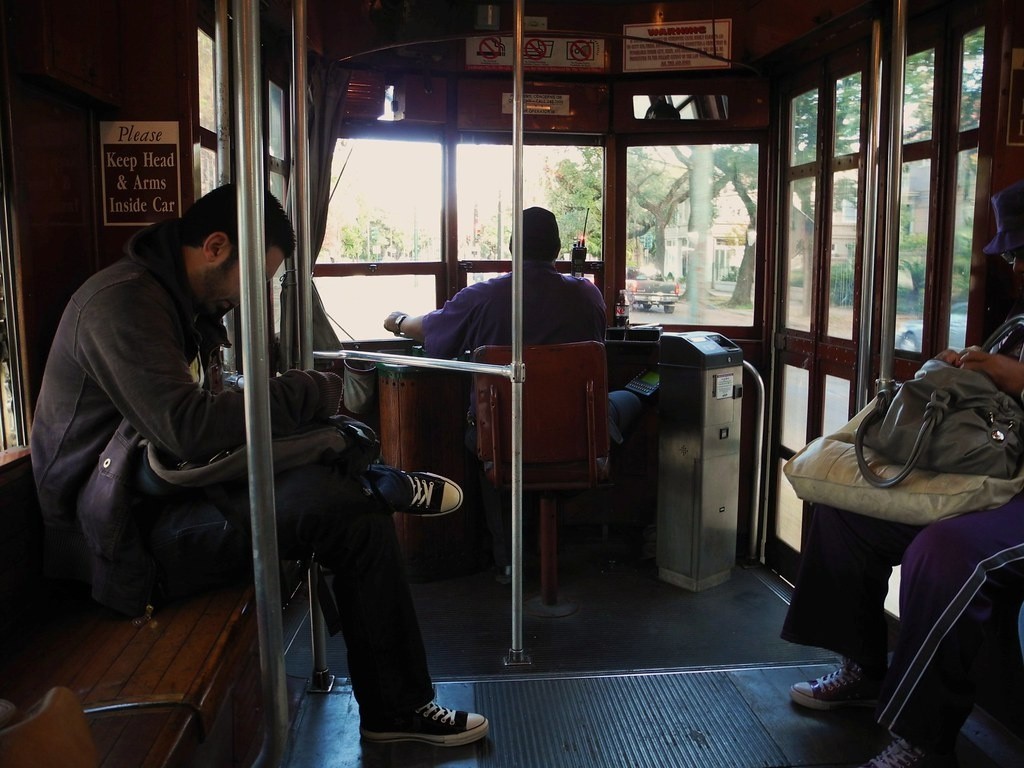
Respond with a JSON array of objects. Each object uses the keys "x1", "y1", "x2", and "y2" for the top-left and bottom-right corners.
[{"x1": 1000, "y1": 248, "x2": 1024, "y2": 265}]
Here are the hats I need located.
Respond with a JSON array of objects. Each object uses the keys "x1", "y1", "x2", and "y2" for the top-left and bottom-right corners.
[
  {"x1": 515, "y1": 208, "x2": 558, "y2": 261},
  {"x1": 983, "y1": 180, "x2": 1024, "y2": 255}
]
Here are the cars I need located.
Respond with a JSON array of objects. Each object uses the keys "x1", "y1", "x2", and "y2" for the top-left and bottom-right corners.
[{"x1": 895, "y1": 301, "x2": 969, "y2": 356}]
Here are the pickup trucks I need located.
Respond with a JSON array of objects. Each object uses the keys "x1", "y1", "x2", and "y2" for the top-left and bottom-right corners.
[{"x1": 626, "y1": 264, "x2": 681, "y2": 313}]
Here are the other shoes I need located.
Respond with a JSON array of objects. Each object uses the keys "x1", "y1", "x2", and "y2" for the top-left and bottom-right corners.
[{"x1": 495, "y1": 564, "x2": 512, "y2": 585}]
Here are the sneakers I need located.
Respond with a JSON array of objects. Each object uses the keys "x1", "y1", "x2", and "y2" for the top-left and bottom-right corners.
[
  {"x1": 788, "y1": 661, "x2": 888, "y2": 710},
  {"x1": 392, "y1": 471, "x2": 463, "y2": 517},
  {"x1": 359, "y1": 701, "x2": 488, "y2": 745},
  {"x1": 857, "y1": 738, "x2": 920, "y2": 768}
]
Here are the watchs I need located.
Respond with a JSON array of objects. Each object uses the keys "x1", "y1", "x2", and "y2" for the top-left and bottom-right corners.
[{"x1": 395, "y1": 315, "x2": 406, "y2": 333}]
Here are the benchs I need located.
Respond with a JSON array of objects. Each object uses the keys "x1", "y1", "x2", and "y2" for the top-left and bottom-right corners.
[{"x1": 0, "y1": 446, "x2": 331, "y2": 768}]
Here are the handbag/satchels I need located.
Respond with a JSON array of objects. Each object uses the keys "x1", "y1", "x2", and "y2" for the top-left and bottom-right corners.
[
  {"x1": 138, "y1": 385, "x2": 378, "y2": 490},
  {"x1": 854, "y1": 314, "x2": 1024, "y2": 488}
]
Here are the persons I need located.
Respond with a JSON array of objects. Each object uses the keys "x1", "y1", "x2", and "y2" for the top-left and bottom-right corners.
[
  {"x1": 382, "y1": 207, "x2": 645, "y2": 583},
  {"x1": 780, "y1": 179, "x2": 1024, "y2": 768},
  {"x1": 30, "y1": 186, "x2": 488, "y2": 745}
]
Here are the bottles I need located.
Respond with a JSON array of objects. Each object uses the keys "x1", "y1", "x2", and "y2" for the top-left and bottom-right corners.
[{"x1": 615, "y1": 290, "x2": 629, "y2": 328}]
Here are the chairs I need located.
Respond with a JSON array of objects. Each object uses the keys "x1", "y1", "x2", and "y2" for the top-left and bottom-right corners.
[{"x1": 473, "y1": 340, "x2": 618, "y2": 617}]
